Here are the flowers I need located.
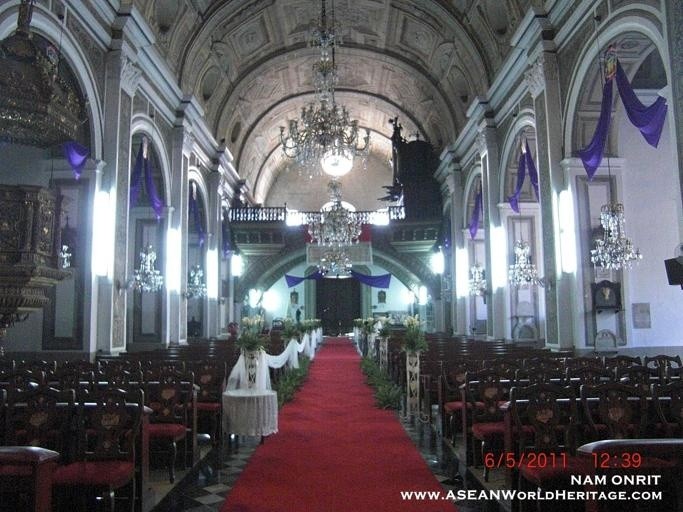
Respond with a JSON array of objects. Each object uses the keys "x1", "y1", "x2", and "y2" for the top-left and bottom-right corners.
[
  {"x1": 234, "y1": 313, "x2": 323, "y2": 351},
  {"x1": 353, "y1": 315, "x2": 431, "y2": 354}
]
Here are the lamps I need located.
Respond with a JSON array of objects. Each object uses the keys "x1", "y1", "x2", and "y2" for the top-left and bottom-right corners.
[
  {"x1": 469, "y1": 244, "x2": 487, "y2": 297},
  {"x1": 508, "y1": 113, "x2": 540, "y2": 285},
  {"x1": 290, "y1": 287, "x2": 298, "y2": 304},
  {"x1": 123, "y1": 140, "x2": 164, "y2": 294},
  {"x1": 278, "y1": 0, "x2": 372, "y2": 279},
  {"x1": 182, "y1": 239, "x2": 208, "y2": 301},
  {"x1": 377, "y1": 291, "x2": 386, "y2": 303},
  {"x1": 590, "y1": 19, "x2": 644, "y2": 270}
]
